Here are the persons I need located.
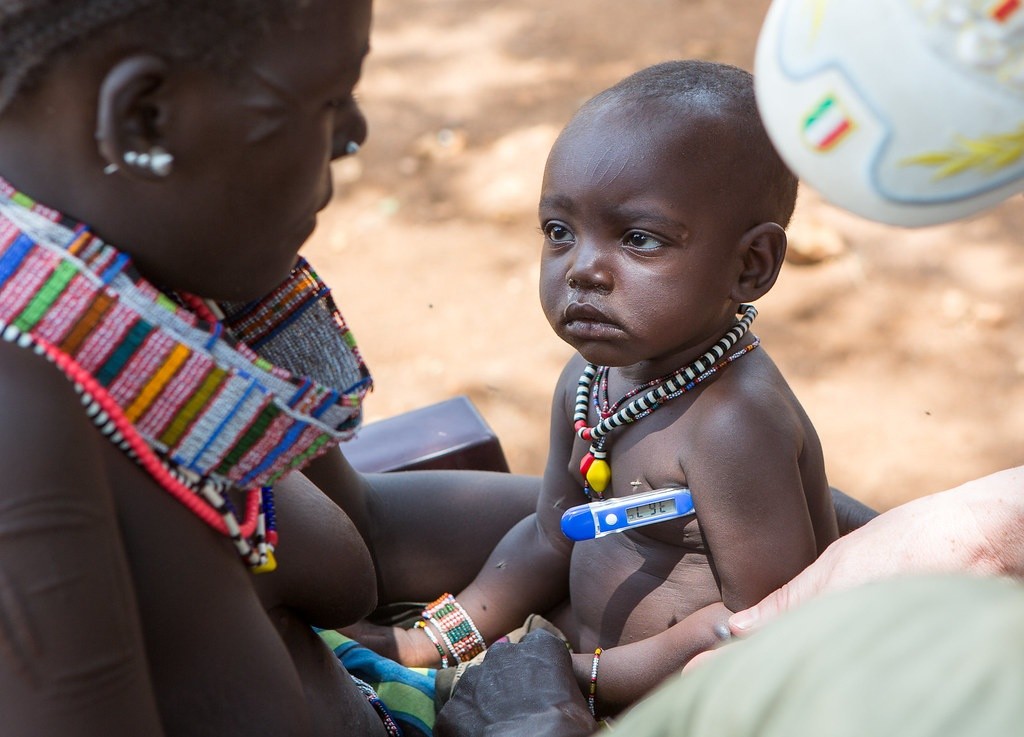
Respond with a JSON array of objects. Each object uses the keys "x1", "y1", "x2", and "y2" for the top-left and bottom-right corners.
[
  {"x1": 341, "y1": 60, "x2": 843, "y2": 680},
  {"x1": 0, "y1": 0, "x2": 886, "y2": 737},
  {"x1": 727, "y1": 463, "x2": 1024, "y2": 640}
]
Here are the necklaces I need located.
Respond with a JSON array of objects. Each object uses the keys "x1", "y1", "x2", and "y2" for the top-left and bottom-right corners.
[
  {"x1": 570, "y1": 302, "x2": 763, "y2": 502},
  {"x1": 0, "y1": 180, "x2": 374, "y2": 577}
]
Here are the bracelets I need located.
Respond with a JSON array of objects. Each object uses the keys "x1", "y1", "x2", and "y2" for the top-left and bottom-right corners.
[
  {"x1": 412, "y1": 587, "x2": 490, "y2": 669},
  {"x1": 587, "y1": 645, "x2": 603, "y2": 720}
]
